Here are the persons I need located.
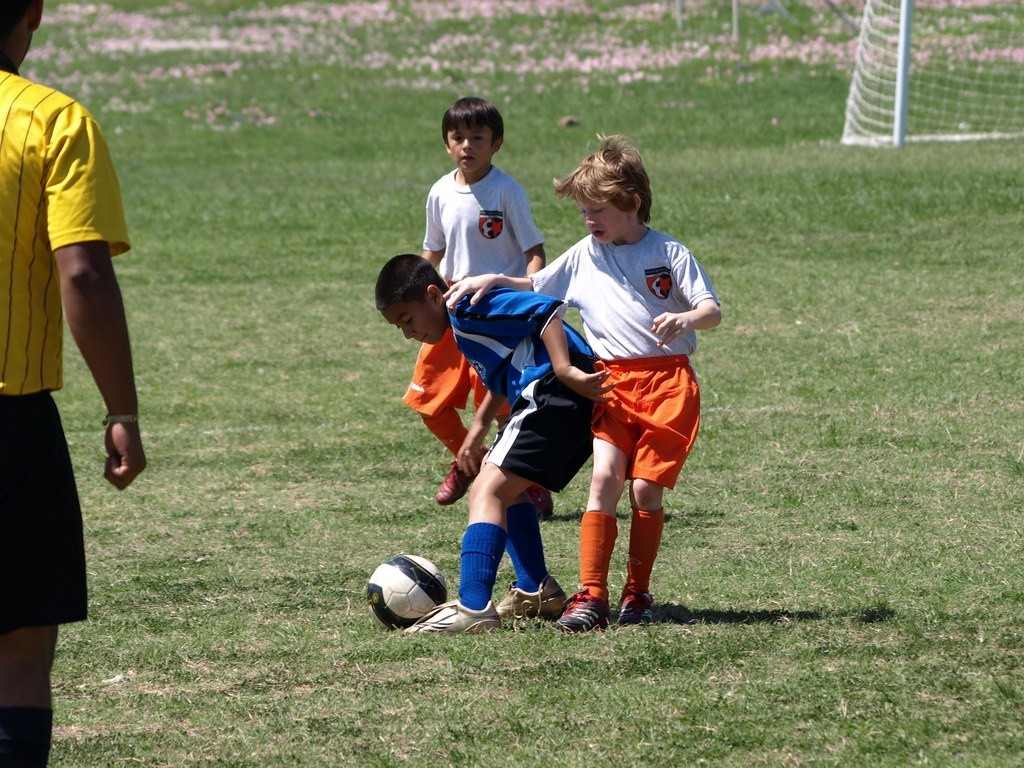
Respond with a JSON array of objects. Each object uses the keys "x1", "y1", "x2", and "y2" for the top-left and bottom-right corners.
[
  {"x1": 0, "y1": 0, "x2": 148, "y2": 768},
  {"x1": 375, "y1": 96, "x2": 721, "y2": 636}
]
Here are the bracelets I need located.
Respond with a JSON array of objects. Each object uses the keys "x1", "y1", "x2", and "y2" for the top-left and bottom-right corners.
[{"x1": 102, "y1": 413, "x2": 139, "y2": 426}]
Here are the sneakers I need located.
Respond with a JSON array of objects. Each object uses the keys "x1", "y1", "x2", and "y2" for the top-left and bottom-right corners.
[
  {"x1": 556, "y1": 586, "x2": 609, "y2": 632},
  {"x1": 495, "y1": 574, "x2": 566, "y2": 619},
  {"x1": 436, "y1": 444, "x2": 490, "y2": 505},
  {"x1": 524, "y1": 483, "x2": 553, "y2": 520},
  {"x1": 616, "y1": 584, "x2": 653, "y2": 625},
  {"x1": 403, "y1": 597, "x2": 502, "y2": 636}
]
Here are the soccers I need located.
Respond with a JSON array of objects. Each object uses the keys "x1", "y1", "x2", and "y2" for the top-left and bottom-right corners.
[{"x1": 367, "y1": 554, "x2": 446, "y2": 630}]
